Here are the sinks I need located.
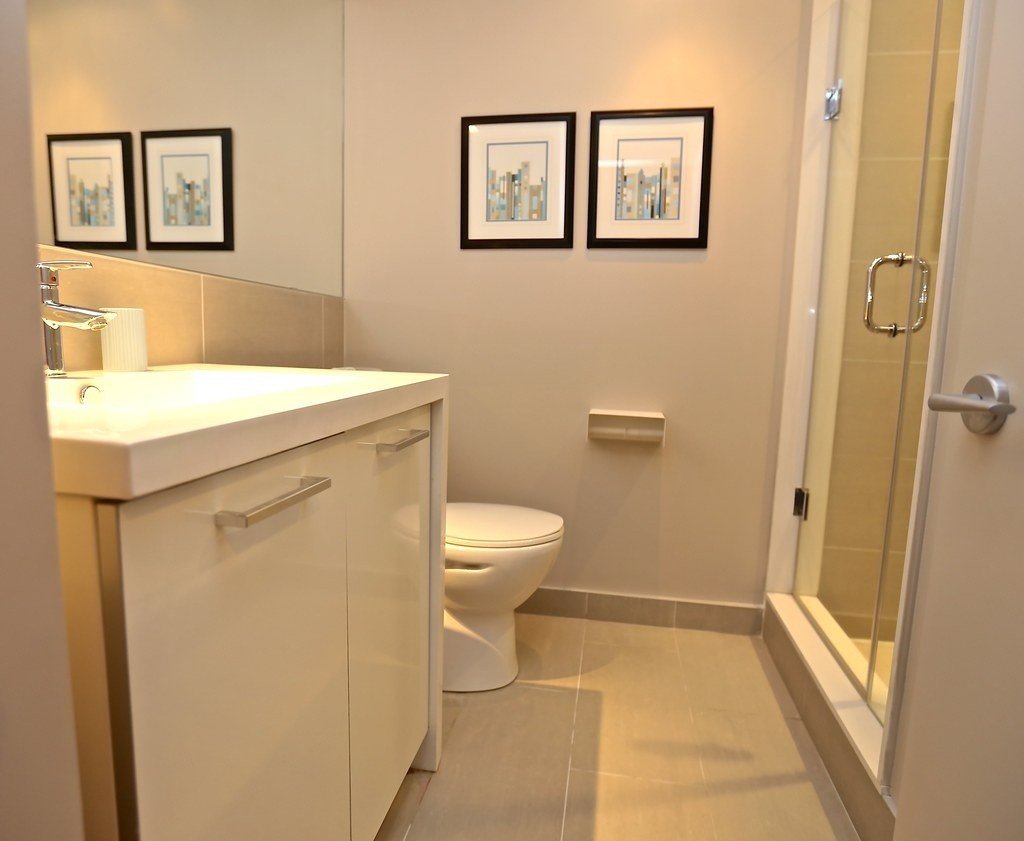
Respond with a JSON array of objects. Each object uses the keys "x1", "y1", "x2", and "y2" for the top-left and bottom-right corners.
[{"x1": 42, "y1": 368, "x2": 387, "y2": 427}]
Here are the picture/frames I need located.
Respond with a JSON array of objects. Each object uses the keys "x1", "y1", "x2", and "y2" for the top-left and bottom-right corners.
[
  {"x1": 140, "y1": 126, "x2": 234, "y2": 250},
  {"x1": 587, "y1": 107, "x2": 714, "y2": 249},
  {"x1": 460, "y1": 110, "x2": 577, "y2": 249},
  {"x1": 45, "y1": 132, "x2": 138, "y2": 252}
]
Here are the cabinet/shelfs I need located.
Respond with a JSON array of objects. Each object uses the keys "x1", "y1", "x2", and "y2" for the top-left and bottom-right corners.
[{"x1": 93, "y1": 400, "x2": 433, "y2": 841}]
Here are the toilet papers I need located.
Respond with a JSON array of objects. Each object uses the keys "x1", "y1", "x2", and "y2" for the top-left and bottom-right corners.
[{"x1": 589, "y1": 424, "x2": 664, "y2": 441}]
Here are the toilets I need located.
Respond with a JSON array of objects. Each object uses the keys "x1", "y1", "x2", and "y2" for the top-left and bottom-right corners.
[{"x1": 439, "y1": 498, "x2": 565, "y2": 695}]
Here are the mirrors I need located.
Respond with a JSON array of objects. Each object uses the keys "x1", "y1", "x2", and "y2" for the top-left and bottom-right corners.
[{"x1": 26, "y1": 1, "x2": 344, "y2": 292}]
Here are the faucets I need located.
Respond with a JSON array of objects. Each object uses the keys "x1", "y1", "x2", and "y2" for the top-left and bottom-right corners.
[{"x1": 38, "y1": 257, "x2": 122, "y2": 376}]
